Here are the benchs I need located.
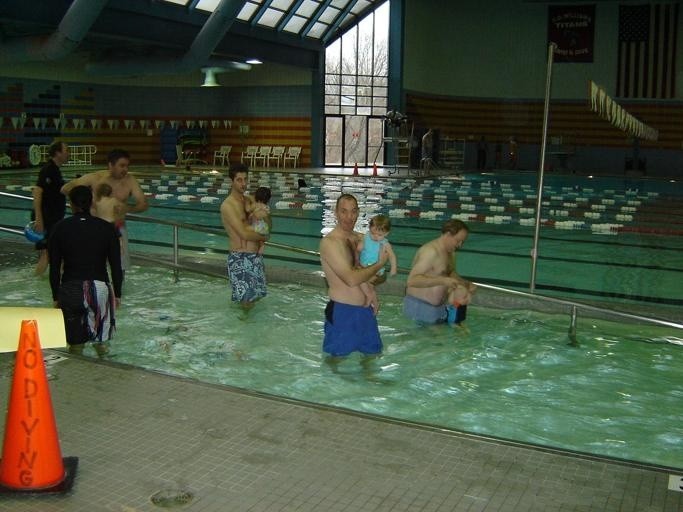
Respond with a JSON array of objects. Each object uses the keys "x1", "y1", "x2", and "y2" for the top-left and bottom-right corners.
[{"x1": 406, "y1": 93, "x2": 683, "y2": 144}]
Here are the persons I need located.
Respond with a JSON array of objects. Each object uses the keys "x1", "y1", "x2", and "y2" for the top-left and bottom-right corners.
[
  {"x1": 421, "y1": 129, "x2": 434, "y2": 175},
  {"x1": 238, "y1": 186, "x2": 272, "y2": 250},
  {"x1": 31, "y1": 139, "x2": 70, "y2": 276},
  {"x1": 91, "y1": 183, "x2": 127, "y2": 252},
  {"x1": 219, "y1": 164, "x2": 273, "y2": 318},
  {"x1": 59, "y1": 148, "x2": 150, "y2": 278},
  {"x1": 318, "y1": 193, "x2": 389, "y2": 371},
  {"x1": 48, "y1": 184, "x2": 124, "y2": 359},
  {"x1": 477, "y1": 135, "x2": 520, "y2": 169},
  {"x1": 353, "y1": 215, "x2": 398, "y2": 316},
  {"x1": 402, "y1": 218, "x2": 478, "y2": 325},
  {"x1": 445, "y1": 284, "x2": 472, "y2": 335}
]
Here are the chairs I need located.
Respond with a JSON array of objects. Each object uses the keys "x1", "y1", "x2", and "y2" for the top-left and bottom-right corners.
[{"x1": 159, "y1": 142, "x2": 302, "y2": 170}]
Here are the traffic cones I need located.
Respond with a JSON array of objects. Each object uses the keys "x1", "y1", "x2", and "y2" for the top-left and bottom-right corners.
[{"x1": 1, "y1": 320, "x2": 77, "y2": 499}]
